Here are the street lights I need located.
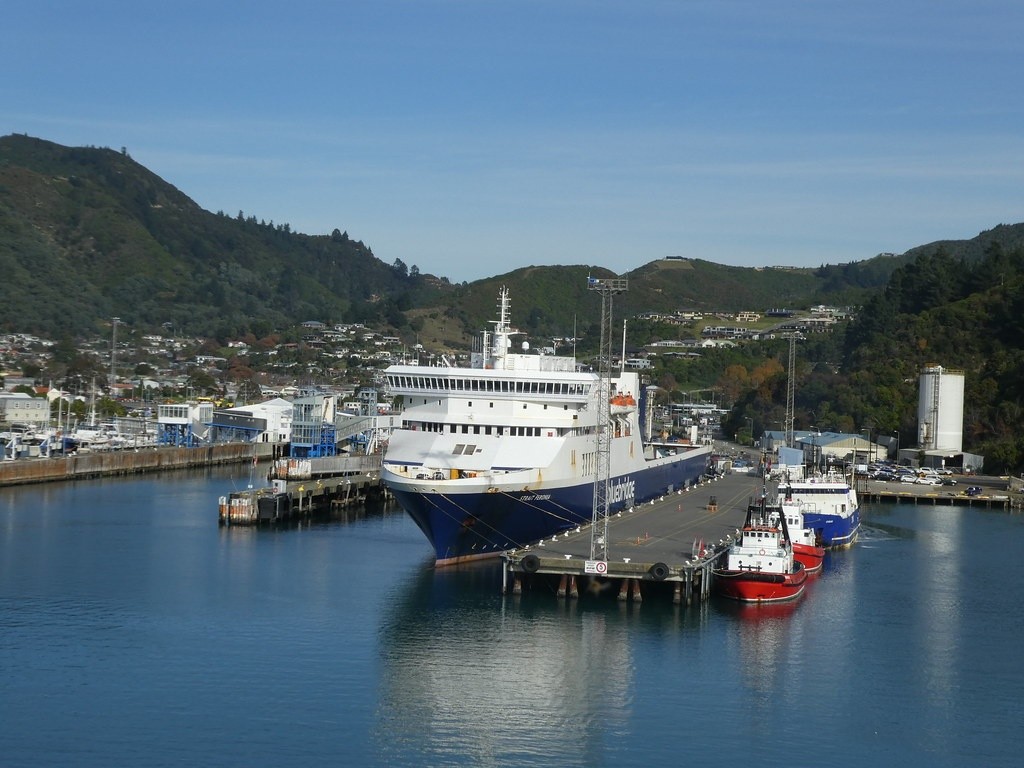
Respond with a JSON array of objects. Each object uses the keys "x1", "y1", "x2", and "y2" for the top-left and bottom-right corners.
[
  {"x1": 862, "y1": 429, "x2": 871, "y2": 464},
  {"x1": 810, "y1": 426, "x2": 819, "y2": 433},
  {"x1": 774, "y1": 422, "x2": 783, "y2": 445},
  {"x1": 895, "y1": 431, "x2": 899, "y2": 469},
  {"x1": 745, "y1": 417, "x2": 753, "y2": 446}
]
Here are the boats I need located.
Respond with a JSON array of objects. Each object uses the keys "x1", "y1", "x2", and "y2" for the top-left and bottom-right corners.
[
  {"x1": 760, "y1": 469, "x2": 825, "y2": 572},
  {"x1": 378, "y1": 285, "x2": 713, "y2": 567},
  {"x1": 776, "y1": 466, "x2": 862, "y2": 551},
  {"x1": 611, "y1": 395, "x2": 636, "y2": 406},
  {"x1": 718, "y1": 451, "x2": 807, "y2": 603}
]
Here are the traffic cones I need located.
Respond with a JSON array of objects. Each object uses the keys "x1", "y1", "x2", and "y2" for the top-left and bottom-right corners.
[
  {"x1": 644, "y1": 533, "x2": 649, "y2": 540},
  {"x1": 636, "y1": 537, "x2": 640, "y2": 544},
  {"x1": 677, "y1": 505, "x2": 681, "y2": 511}
]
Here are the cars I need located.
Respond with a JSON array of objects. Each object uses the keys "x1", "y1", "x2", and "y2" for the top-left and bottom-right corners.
[
  {"x1": 941, "y1": 478, "x2": 957, "y2": 486},
  {"x1": 936, "y1": 468, "x2": 953, "y2": 475},
  {"x1": 965, "y1": 487, "x2": 982, "y2": 495},
  {"x1": 915, "y1": 477, "x2": 935, "y2": 486},
  {"x1": 868, "y1": 464, "x2": 942, "y2": 484}
]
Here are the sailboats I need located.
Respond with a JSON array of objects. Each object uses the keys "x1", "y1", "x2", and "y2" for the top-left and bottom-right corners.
[{"x1": 0, "y1": 377, "x2": 158, "y2": 459}]
